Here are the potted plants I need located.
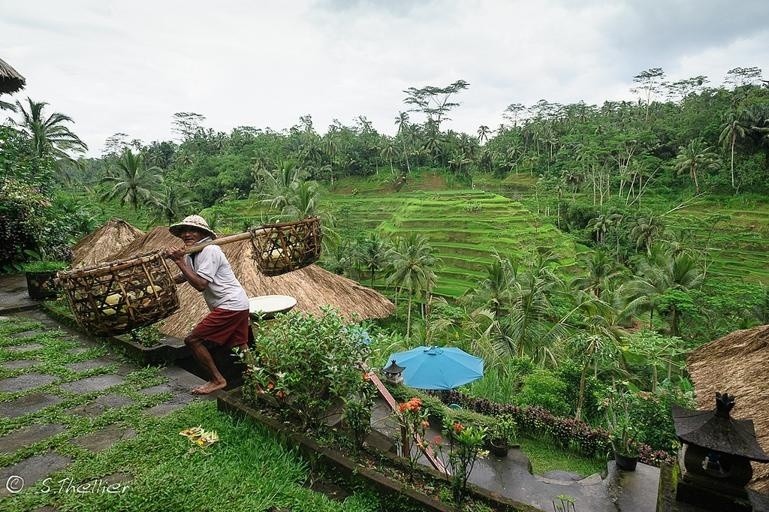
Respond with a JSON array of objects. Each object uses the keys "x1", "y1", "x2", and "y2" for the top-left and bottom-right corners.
[
  {"x1": 595, "y1": 386, "x2": 664, "y2": 472},
  {"x1": 490, "y1": 412, "x2": 518, "y2": 459},
  {"x1": 20, "y1": 260, "x2": 62, "y2": 300}
]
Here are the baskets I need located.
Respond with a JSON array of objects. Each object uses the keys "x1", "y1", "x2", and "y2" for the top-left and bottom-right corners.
[
  {"x1": 57, "y1": 250, "x2": 180, "y2": 336},
  {"x1": 250, "y1": 216, "x2": 322, "y2": 277}
]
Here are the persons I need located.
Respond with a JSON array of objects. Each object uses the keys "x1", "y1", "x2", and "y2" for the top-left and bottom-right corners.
[{"x1": 163, "y1": 214, "x2": 253, "y2": 393}]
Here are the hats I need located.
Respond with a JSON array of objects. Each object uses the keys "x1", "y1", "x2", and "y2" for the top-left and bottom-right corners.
[{"x1": 169, "y1": 215, "x2": 217, "y2": 240}]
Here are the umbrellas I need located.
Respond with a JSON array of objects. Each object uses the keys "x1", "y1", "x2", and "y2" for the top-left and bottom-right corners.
[{"x1": 380, "y1": 343, "x2": 486, "y2": 391}]
